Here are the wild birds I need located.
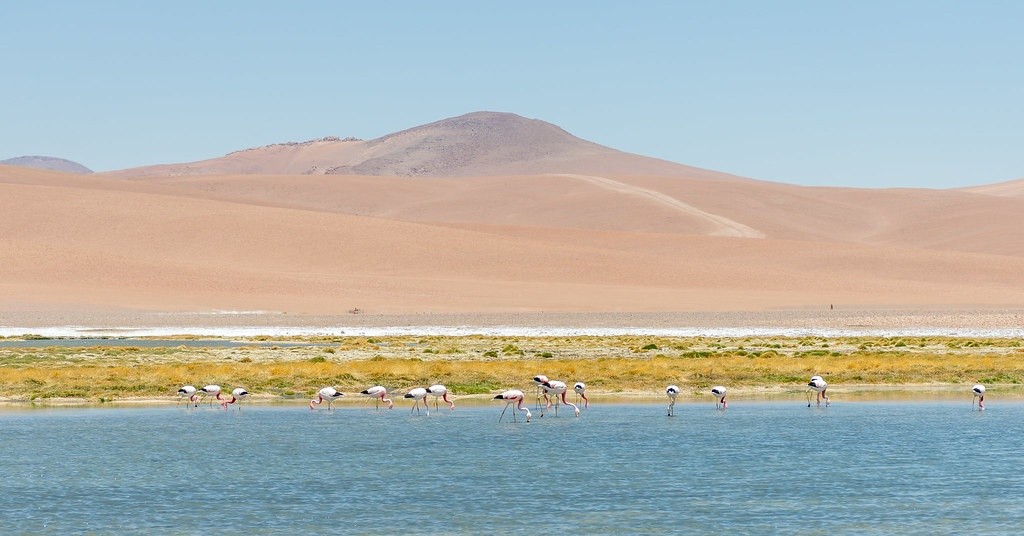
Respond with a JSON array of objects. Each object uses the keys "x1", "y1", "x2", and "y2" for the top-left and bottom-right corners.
[
  {"x1": 425, "y1": 385, "x2": 455, "y2": 412},
  {"x1": 574, "y1": 382, "x2": 588, "y2": 408},
  {"x1": 972, "y1": 384, "x2": 985, "y2": 407},
  {"x1": 537, "y1": 381, "x2": 580, "y2": 417},
  {"x1": 711, "y1": 386, "x2": 727, "y2": 410},
  {"x1": 533, "y1": 375, "x2": 552, "y2": 410},
  {"x1": 225, "y1": 387, "x2": 250, "y2": 410},
  {"x1": 666, "y1": 385, "x2": 680, "y2": 416},
  {"x1": 494, "y1": 390, "x2": 532, "y2": 423},
  {"x1": 405, "y1": 388, "x2": 430, "y2": 416},
  {"x1": 806, "y1": 375, "x2": 830, "y2": 407},
  {"x1": 176, "y1": 386, "x2": 197, "y2": 409},
  {"x1": 197, "y1": 385, "x2": 224, "y2": 408},
  {"x1": 361, "y1": 386, "x2": 393, "y2": 410},
  {"x1": 310, "y1": 386, "x2": 344, "y2": 410}
]
